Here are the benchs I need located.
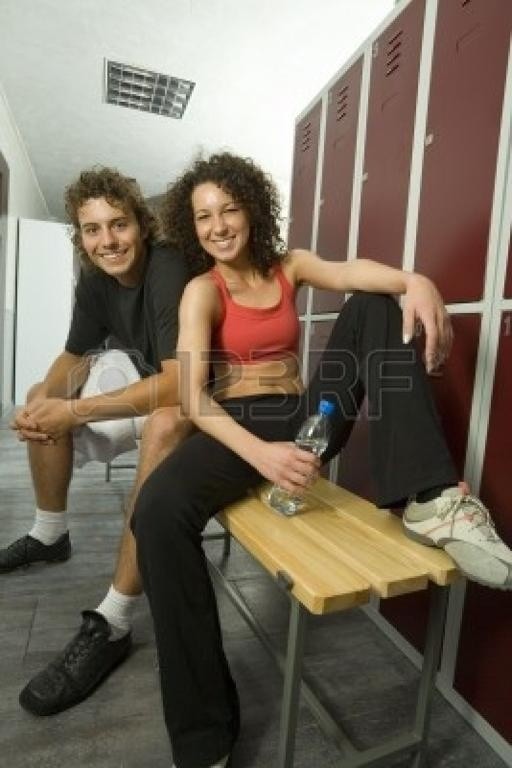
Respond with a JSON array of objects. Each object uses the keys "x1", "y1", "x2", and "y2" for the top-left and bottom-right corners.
[{"x1": 105, "y1": 442, "x2": 462, "y2": 767}]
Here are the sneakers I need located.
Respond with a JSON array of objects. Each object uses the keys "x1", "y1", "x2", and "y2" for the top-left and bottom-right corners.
[
  {"x1": 19, "y1": 610, "x2": 134, "y2": 716},
  {"x1": 402, "y1": 479, "x2": 512, "y2": 594},
  {"x1": 0, "y1": 529, "x2": 73, "y2": 574}
]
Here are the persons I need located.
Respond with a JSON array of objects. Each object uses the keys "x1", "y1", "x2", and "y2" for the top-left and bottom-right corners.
[
  {"x1": 129, "y1": 151, "x2": 512, "y2": 768},
  {"x1": 0, "y1": 166, "x2": 198, "y2": 716}
]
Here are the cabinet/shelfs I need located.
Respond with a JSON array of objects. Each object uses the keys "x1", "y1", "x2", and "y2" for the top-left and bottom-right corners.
[{"x1": 286, "y1": 1, "x2": 508, "y2": 760}]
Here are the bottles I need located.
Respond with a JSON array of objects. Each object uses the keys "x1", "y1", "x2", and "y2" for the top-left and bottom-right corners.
[{"x1": 270, "y1": 401, "x2": 334, "y2": 518}]
